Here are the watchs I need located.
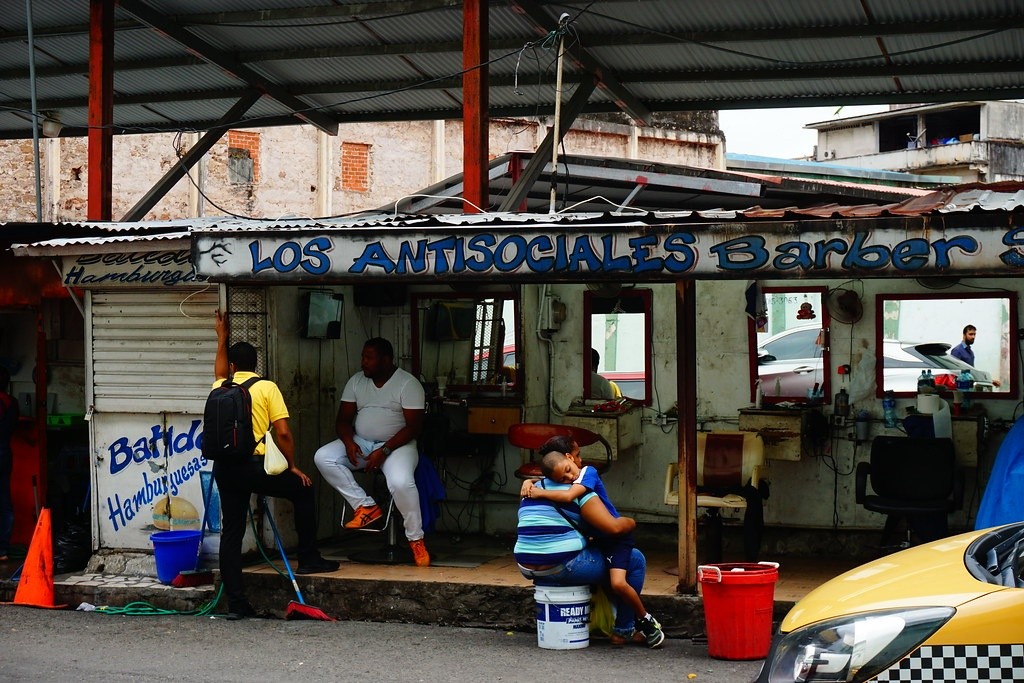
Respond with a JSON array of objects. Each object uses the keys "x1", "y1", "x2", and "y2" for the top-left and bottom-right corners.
[{"x1": 381, "y1": 444, "x2": 392, "y2": 456}]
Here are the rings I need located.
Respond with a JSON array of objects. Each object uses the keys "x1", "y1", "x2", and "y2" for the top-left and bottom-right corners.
[{"x1": 374, "y1": 467, "x2": 377, "y2": 469}]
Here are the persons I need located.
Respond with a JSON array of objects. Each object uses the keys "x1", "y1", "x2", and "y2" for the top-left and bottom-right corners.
[
  {"x1": 313, "y1": 338, "x2": 430, "y2": 567},
  {"x1": 591, "y1": 348, "x2": 613, "y2": 399},
  {"x1": 513, "y1": 436, "x2": 646, "y2": 643},
  {"x1": 212, "y1": 311, "x2": 339, "y2": 621},
  {"x1": 0, "y1": 365, "x2": 20, "y2": 561},
  {"x1": 520, "y1": 451, "x2": 664, "y2": 648},
  {"x1": 951, "y1": 325, "x2": 976, "y2": 367}
]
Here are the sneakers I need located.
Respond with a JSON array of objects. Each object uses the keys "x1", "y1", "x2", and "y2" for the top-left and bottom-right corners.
[
  {"x1": 638, "y1": 613, "x2": 664, "y2": 648},
  {"x1": 297, "y1": 556, "x2": 340, "y2": 574},
  {"x1": 410, "y1": 539, "x2": 429, "y2": 568},
  {"x1": 226, "y1": 597, "x2": 269, "y2": 620},
  {"x1": 344, "y1": 505, "x2": 381, "y2": 529}
]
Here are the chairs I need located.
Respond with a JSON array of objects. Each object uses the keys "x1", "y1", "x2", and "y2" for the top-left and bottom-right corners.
[
  {"x1": 665, "y1": 430, "x2": 771, "y2": 574},
  {"x1": 509, "y1": 424, "x2": 612, "y2": 481},
  {"x1": 856, "y1": 435, "x2": 967, "y2": 556},
  {"x1": 339, "y1": 420, "x2": 429, "y2": 564}
]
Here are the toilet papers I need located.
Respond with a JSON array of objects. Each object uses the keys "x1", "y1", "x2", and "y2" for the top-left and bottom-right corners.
[{"x1": 917, "y1": 393, "x2": 940, "y2": 413}]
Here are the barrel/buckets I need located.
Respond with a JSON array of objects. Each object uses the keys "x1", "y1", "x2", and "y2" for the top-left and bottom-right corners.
[
  {"x1": 150, "y1": 530, "x2": 203, "y2": 585},
  {"x1": 696, "y1": 561, "x2": 781, "y2": 661},
  {"x1": 533, "y1": 578, "x2": 593, "y2": 651}
]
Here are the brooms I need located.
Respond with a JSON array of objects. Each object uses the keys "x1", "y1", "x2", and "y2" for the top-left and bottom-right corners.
[
  {"x1": 170, "y1": 461, "x2": 214, "y2": 588},
  {"x1": 256, "y1": 494, "x2": 337, "y2": 621}
]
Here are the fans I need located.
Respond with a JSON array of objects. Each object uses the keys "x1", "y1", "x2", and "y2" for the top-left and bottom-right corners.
[{"x1": 825, "y1": 288, "x2": 865, "y2": 324}]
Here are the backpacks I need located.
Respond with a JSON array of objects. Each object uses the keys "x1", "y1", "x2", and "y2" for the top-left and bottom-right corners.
[{"x1": 201, "y1": 373, "x2": 271, "y2": 464}]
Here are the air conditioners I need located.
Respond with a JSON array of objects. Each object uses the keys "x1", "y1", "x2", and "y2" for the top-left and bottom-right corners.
[{"x1": 824, "y1": 150, "x2": 835, "y2": 160}]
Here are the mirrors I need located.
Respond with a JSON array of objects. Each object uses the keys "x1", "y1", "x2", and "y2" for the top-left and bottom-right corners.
[
  {"x1": 581, "y1": 287, "x2": 654, "y2": 405},
  {"x1": 745, "y1": 286, "x2": 831, "y2": 405},
  {"x1": 468, "y1": 297, "x2": 519, "y2": 392},
  {"x1": 876, "y1": 294, "x2": 1018, "y2": 398}
]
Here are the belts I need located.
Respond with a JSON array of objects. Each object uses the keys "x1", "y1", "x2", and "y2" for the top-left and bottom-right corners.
[{"x1": 518, "y1": 562, "x2": 572, "y2": 576}]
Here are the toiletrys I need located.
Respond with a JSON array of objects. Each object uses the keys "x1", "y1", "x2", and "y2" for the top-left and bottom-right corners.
[
  {"x1": 755, "y1": 378, "x2": 764, "y2": 408},
  {"x1": 775, "y1": 376, "x2": 783, "y2": 396}
]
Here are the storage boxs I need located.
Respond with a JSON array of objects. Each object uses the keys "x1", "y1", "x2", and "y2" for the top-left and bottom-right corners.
[{"x1": 960, "y1": 134, "x2": 973, "y2": 142}]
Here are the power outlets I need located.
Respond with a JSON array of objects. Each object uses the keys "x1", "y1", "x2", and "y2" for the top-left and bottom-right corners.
[
  {"x1": 835, "y1": 415, "x2": 845, "y2": 426},
  {"x1": 651, "y1": 414, "x2": 667, "y2": 426}
]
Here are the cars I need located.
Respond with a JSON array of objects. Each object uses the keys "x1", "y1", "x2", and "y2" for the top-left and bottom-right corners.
[
  {"x1": 600, "y1": 371, "x2": 644, "y2": 399},
  {"x1": 473, "y1": 344, "x2": 516, "y2": 381},
  {"x1": 751, "y1": 519, "x2": 1024, "y2": 683},
  {"x1": 758, "y1": 323, "x2": 824, "y2": 397},
  {"x1": 883, "y1": 339, "x2": 1000, "y2": 393}
]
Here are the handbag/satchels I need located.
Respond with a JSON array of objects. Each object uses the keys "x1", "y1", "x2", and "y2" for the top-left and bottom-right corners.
[{"x1": 264, "y1": 430, "x2": 288, "y2": 476}]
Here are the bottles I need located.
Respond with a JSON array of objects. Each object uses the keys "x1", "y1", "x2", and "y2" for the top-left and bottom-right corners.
[
  {"x1": 927, "y1": 370, "x2": 935, "y2": 392},
  {"x1": 918, "y1": 370, "x2": 931, "y2": 394},
  {"x1": 477, "y1": 374, "x2": 507, "y2": 396},
  {"x1": 882, "y1": 391, "x2": 897, "y2": 428},
  {"x1": 965, "y1": 369, "x2": 974, "y2": 391},
  {"x1": 834, "y1": 386, "x2": 849, "y2": 416},
  {"x1": 444, "y1": 371, "x2": 450, "y2": 384},
  {"x1": 957, "y1": 369, "x2": 970, "y2": 413}
]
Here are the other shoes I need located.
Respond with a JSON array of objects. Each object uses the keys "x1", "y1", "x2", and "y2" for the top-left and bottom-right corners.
[{"x1": 611, "y1": 630, "x2": 646, "y2": 646}]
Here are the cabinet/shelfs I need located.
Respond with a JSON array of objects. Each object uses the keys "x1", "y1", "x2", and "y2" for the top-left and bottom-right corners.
[
  {"x1": 555, "y1": 409, "x2": 643, "y2": 459},
  {"x1": 464, "y1": 398, "x2": 523, "y2": 432},
  {"x1": 740, "y1": 410, "x2": 829, "y2": 461},
  {"x1": 951, "y1": 416, "x2": 989, "y2": 471}
]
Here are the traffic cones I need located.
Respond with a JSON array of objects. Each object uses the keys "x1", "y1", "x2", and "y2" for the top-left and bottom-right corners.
[{"x1": 0, "y1": 506, "x2": 67, "y2": 608}]
